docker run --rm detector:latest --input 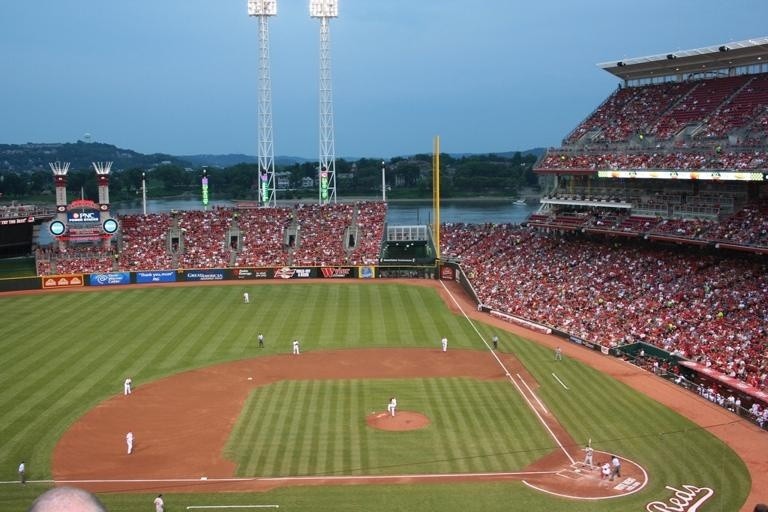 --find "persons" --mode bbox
[583,444,595,471]
[153,493,165,512]
[124,375,132,396]
[386,393,397,418]
[753,502,768,512]
[125,428,134,456]
[258,332,264,349]
[18,461,25,485]
[293,340,301,356]
[597,455,621,480]
[242,290,249,304]
[28,485,107,512]
